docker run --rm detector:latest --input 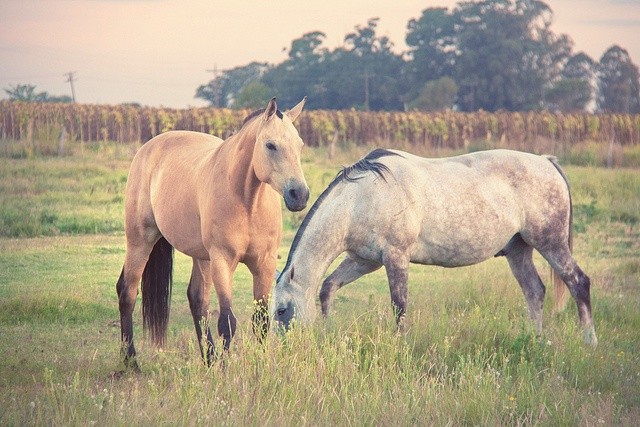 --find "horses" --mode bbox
[116,95,309,378]
[269,149,600,354]
